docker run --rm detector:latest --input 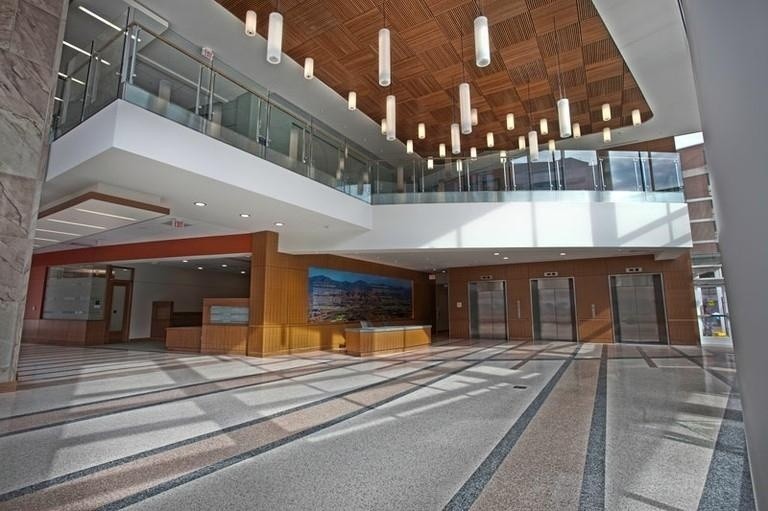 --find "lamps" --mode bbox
[468,106,494,161]
[602,88,613,144]
[418,110,425,139]
[500,108,526,163]
[553,16,573,138]
[473,1,491,67]
[406,123,413,155]
[572,104,582,140]
[632,89,641,128]
[303,30,314,80]
[449,79,460,154]
[381,103,387,135]
[458,29,472,135]
[266,2,284,65]
[539,116,555,152]
[528,78,541,162]
[245,1,256,36]
[348,75,356,110]
[386,82,397,141]
[379,2,391,86]
[427,143,446,168]
